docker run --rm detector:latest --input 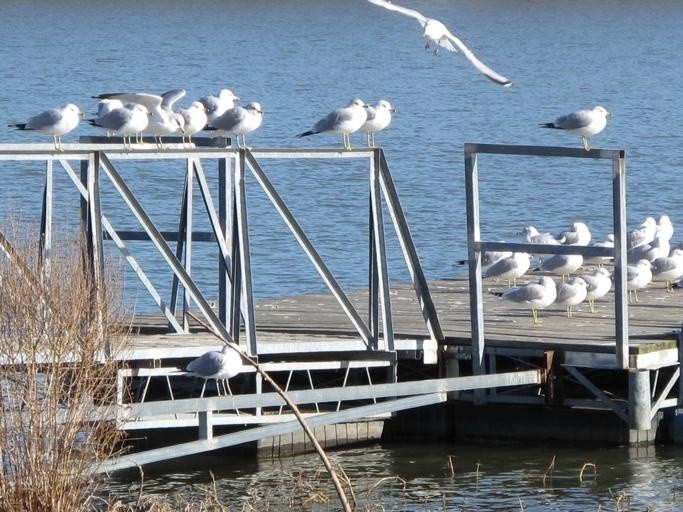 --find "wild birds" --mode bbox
[294,98,369,151]
[368,0,512,88]
[454,215,683,324]
[538,105,613,154]
[80,88,263,153]
[7,103,84,152]
[356,99,396,147]
